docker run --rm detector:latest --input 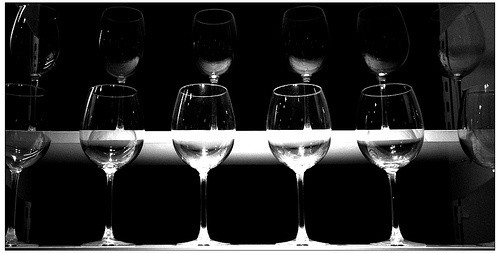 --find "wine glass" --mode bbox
[192,8,238,135]
[456,83,495,246]
[266,83,332,246]
[4,82,56,248]
[431,3,485,141]
[356,83,428,247]
[356,4,410,138]
[9,4,61,133]
[79,85,145,246]
[97,7,144,134]
[170,83,236,247]
[283,5,329,135]
